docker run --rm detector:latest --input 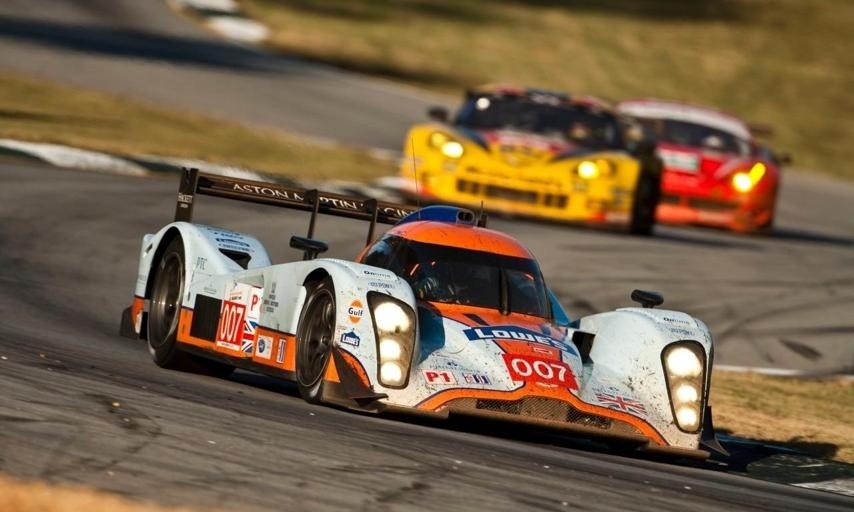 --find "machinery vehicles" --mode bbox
[117,165,732,470]
[398,85,660,238]
[616,98,792,237]
